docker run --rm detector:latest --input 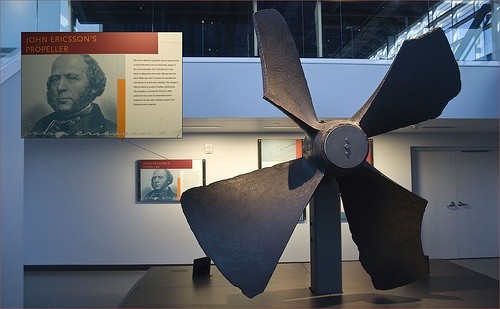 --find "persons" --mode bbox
[145,169,179,201]
[30,53,119,137]
